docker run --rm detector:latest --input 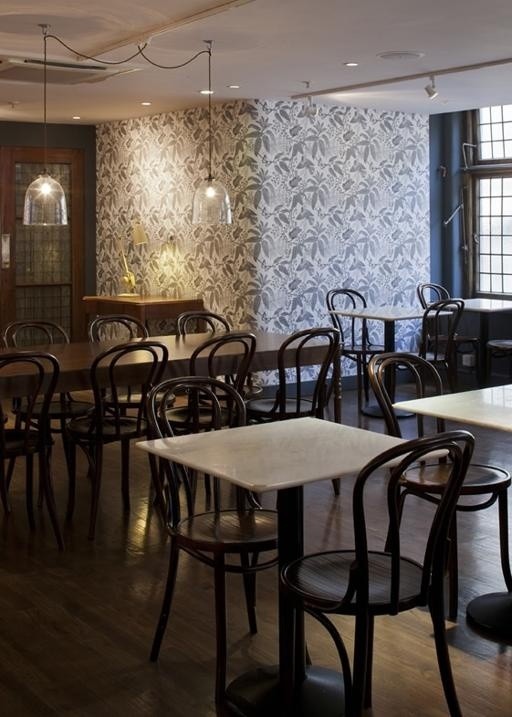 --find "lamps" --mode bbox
[137,40,232,226]
[290,58,512,116]
[117,225,148,297]
[22,24,152,227]
